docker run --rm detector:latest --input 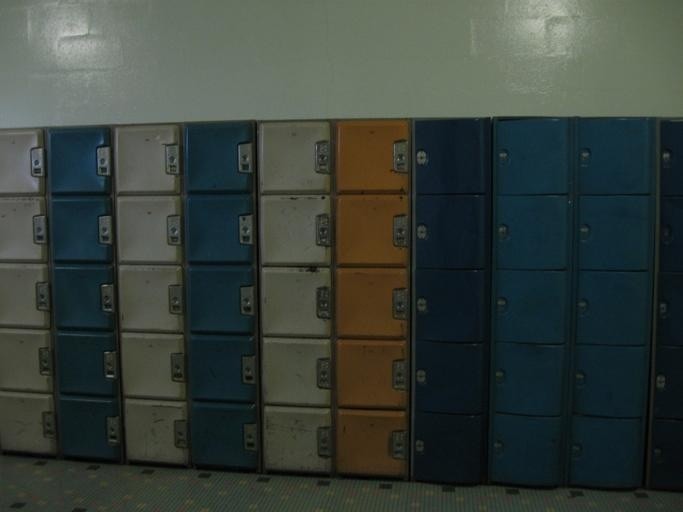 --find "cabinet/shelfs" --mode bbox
[409,114,493,487]
[255,118,335,477]
[645,114,682,494]
[181,119,262,472]
[489,115,579,489]
[565,116,660,490]
[112,122,191,468]
[331,117,413,480]
[1,126,57,457]
[45,125,126,465]
